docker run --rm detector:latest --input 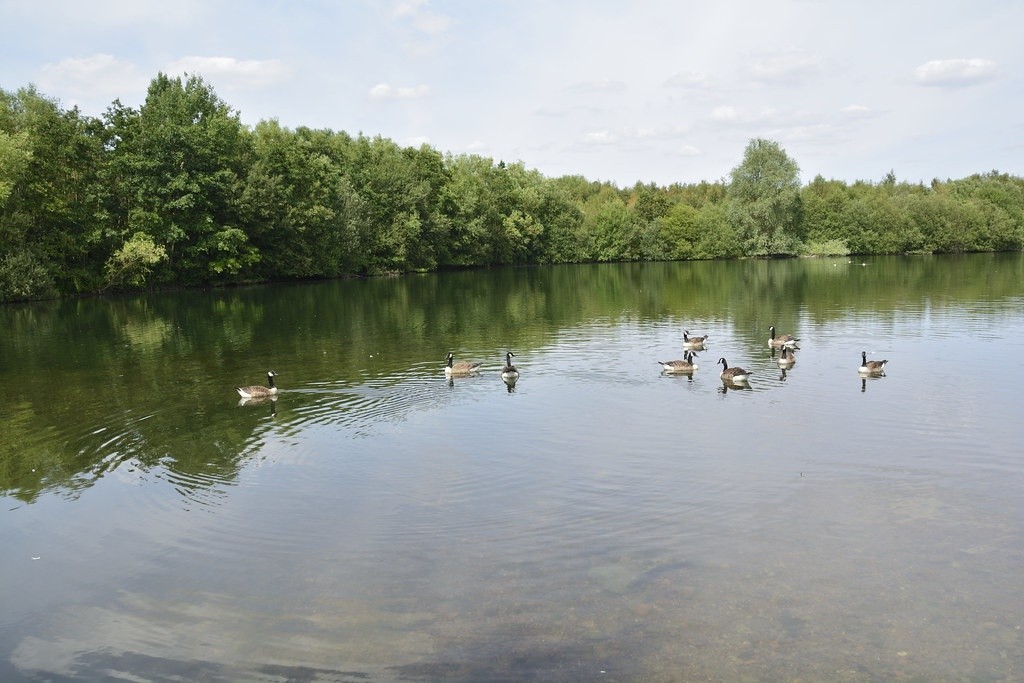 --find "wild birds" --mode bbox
[858,350,888,372]
[658,350,699,371]
[233,369,279,398]
[682,330,709,351]
[777,344,796,364]
[444,351,483,373]
[501,352,519,378]
[717,358,753,380]
[767,325,801,345]
